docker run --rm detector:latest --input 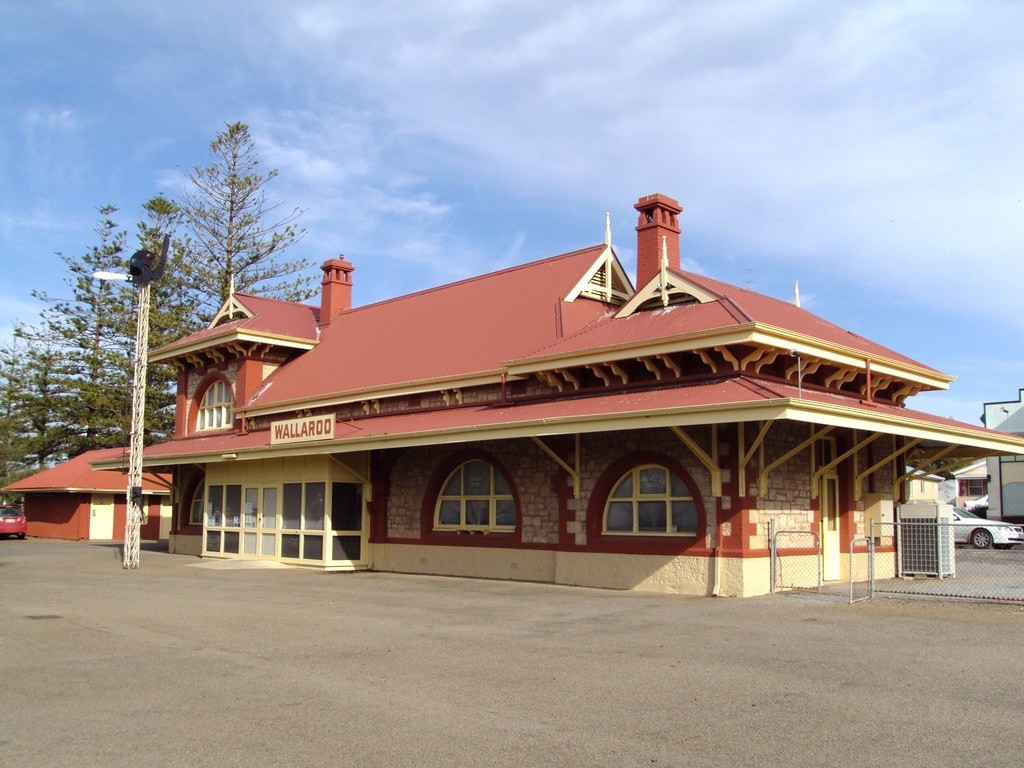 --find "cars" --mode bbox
[964,494,988,512]
[0,505,27,540]
[947,506,1024,550]
